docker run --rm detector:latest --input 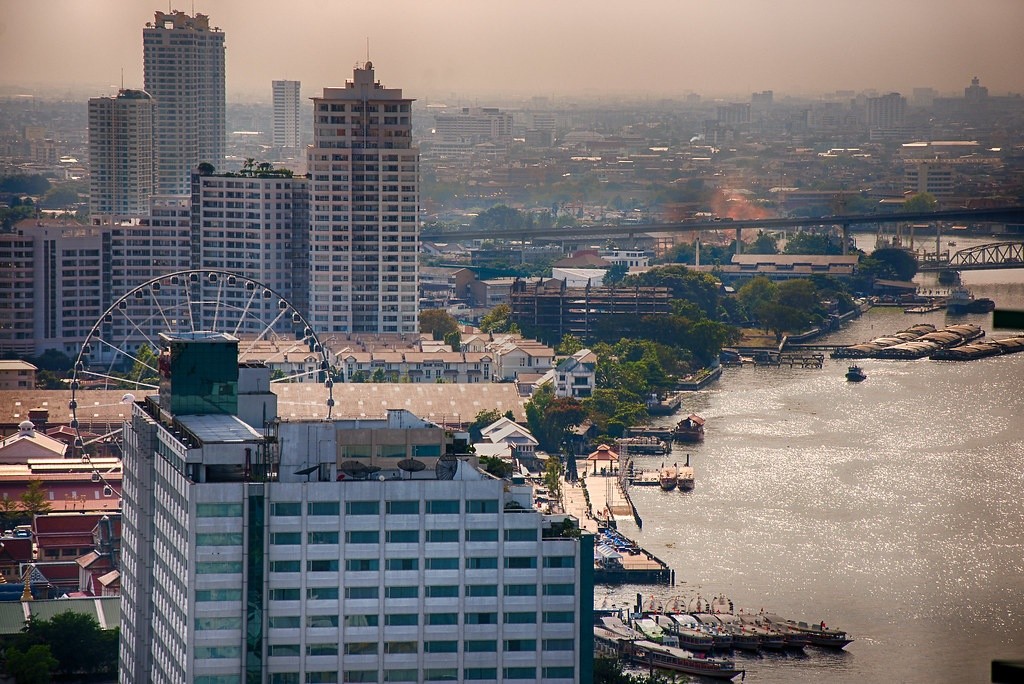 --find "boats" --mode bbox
[661,463,678,491]
[677,454,695,493]
[845,364,866,382]
[593,593,855,677]
[969,298,995,314]
[674,413,706,443]
[945,287,974,312]
[938,271,961,285]
